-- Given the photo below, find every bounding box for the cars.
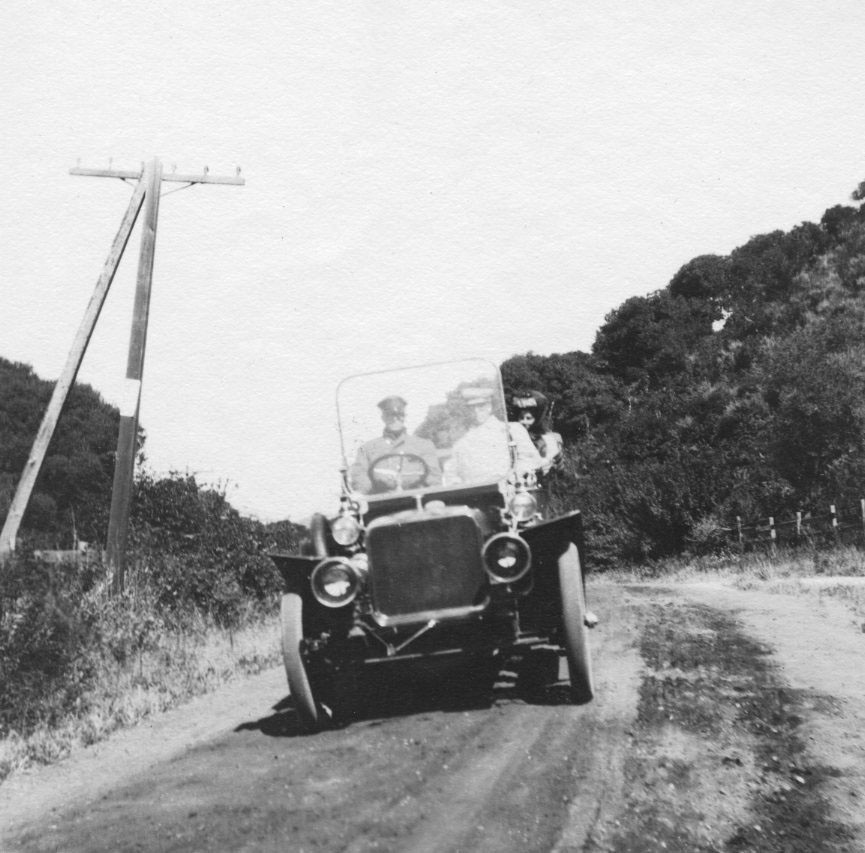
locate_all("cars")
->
[274,357,594,719]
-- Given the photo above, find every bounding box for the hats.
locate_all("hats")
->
[461,387,493,405]
[510,389,548,408]
[378,395,408,410]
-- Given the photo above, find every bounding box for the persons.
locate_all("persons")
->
[440,384,562,491]
[353,397,442,497]
